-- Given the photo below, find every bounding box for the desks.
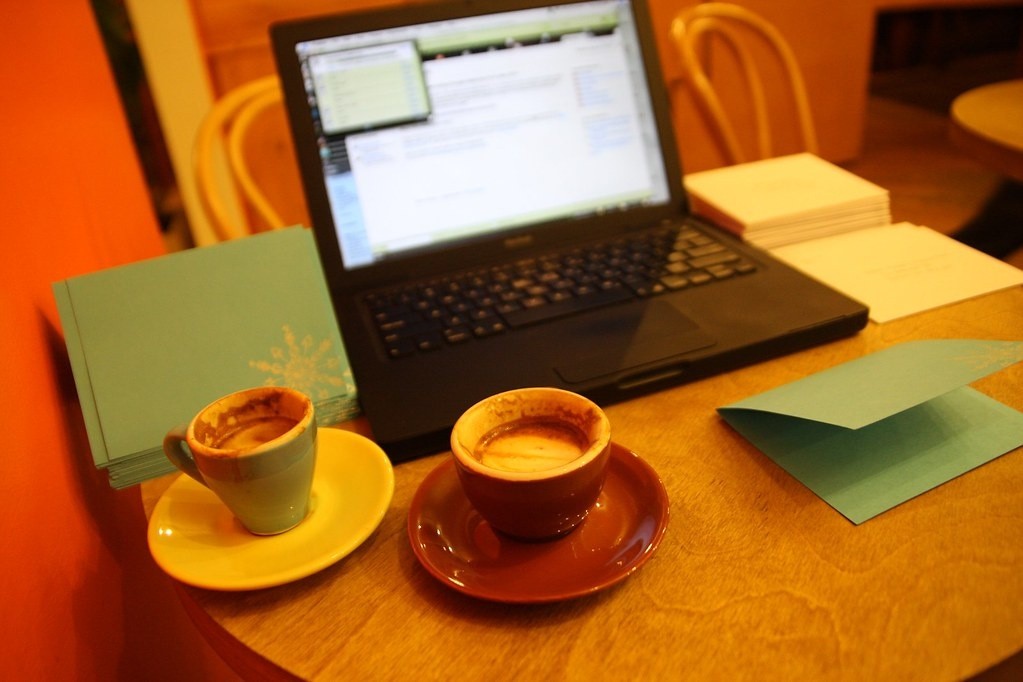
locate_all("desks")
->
[949,79,1023,181]
[137,287,1022,682]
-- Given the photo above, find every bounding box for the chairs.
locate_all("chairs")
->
[669,2,818,165]
[192,74,287,240]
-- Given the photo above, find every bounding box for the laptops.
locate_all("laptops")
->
[270,0,868,472]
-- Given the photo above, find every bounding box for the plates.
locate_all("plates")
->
[406,442,671,604]
[145,427,394,591]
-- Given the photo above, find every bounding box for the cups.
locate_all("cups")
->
[452,387,611,542]
[163,386,318,535]
